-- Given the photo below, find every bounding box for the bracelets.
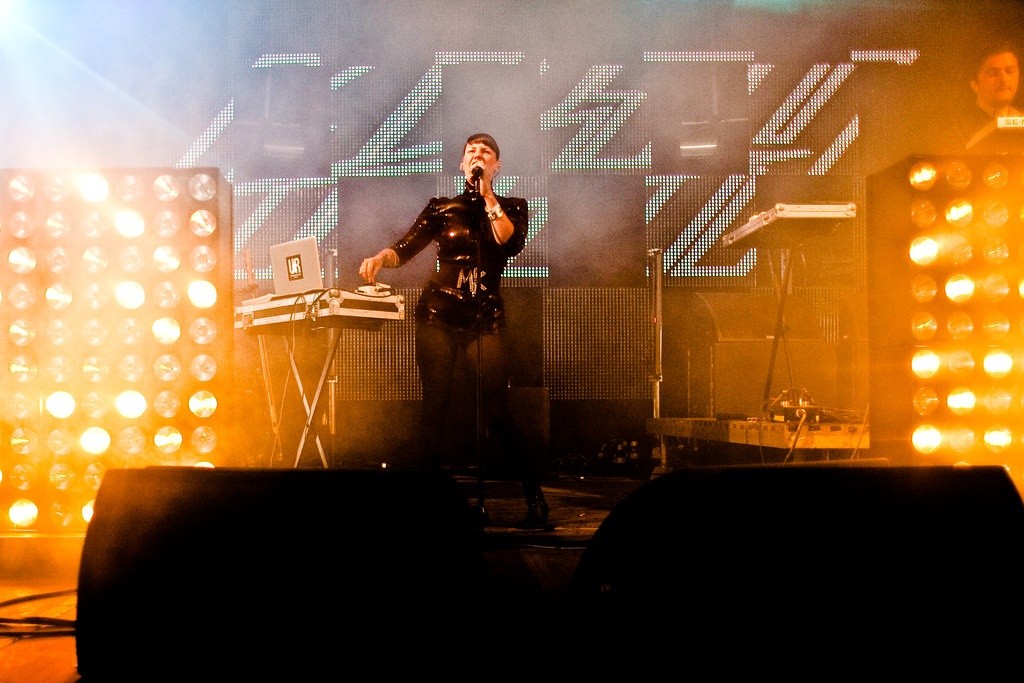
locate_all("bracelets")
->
[391,250,399,267]
[484,204,503,220]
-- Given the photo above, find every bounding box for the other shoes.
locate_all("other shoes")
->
[521,495,550,527]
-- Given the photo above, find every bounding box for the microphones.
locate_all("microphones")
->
[471,166,483,182]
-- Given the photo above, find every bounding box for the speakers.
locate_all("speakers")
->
[76,465,489,683]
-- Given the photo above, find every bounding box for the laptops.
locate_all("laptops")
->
[270,236,324,297]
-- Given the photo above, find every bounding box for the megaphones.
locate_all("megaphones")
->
[577,458,1024,683]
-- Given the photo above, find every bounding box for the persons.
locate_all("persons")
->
[358,132,551,529]
